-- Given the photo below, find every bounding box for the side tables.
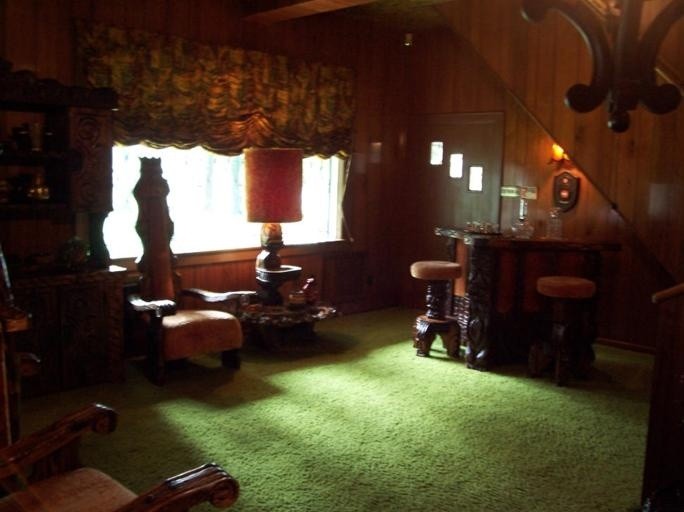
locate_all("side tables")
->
[239,298,344,358]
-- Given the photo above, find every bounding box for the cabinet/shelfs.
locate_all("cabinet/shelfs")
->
[0,53,129,400]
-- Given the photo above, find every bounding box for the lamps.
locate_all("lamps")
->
[243,147,303,307]
[547,143,570,170]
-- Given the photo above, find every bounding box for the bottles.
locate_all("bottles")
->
[510,186,535,240]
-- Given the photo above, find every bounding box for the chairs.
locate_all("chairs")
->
[0,401,240,512]
[123,157,251,388]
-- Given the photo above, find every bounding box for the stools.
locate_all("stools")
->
[528,275,598,387]
[408,260,462,358]
